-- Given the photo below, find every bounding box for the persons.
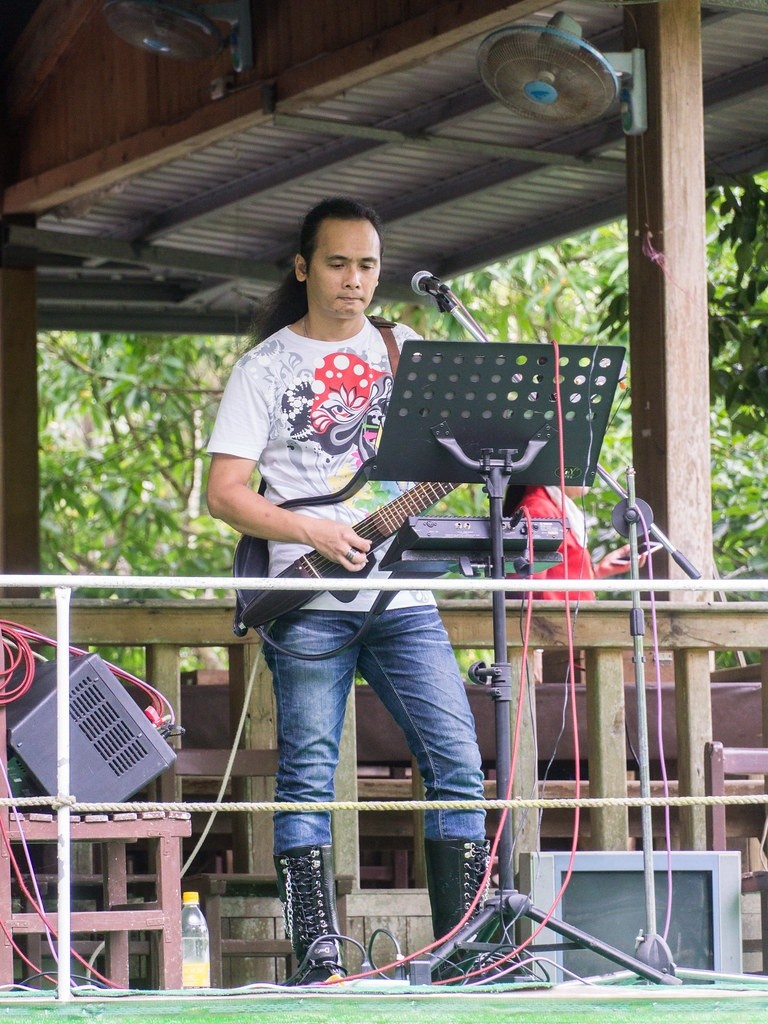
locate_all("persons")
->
[206,197,532,984]
[500,471,645,602]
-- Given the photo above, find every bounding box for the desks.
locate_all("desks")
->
[0,599,768,977]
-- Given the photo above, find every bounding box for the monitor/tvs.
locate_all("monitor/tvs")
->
[514,852,743,984]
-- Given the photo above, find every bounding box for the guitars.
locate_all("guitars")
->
[232,358,629,661]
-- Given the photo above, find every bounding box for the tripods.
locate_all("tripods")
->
[369,276,767,990]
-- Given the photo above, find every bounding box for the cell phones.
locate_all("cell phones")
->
[611,540,663,565]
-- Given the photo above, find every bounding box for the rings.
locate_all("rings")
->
[344,547,356,561]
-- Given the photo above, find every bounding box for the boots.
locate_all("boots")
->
[424,838,511,981]
[270,844,352,983]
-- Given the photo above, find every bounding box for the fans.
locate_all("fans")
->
[474,12,651,138]
[100,0,280,119]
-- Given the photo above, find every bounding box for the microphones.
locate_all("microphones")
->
[410,270,449,295]
